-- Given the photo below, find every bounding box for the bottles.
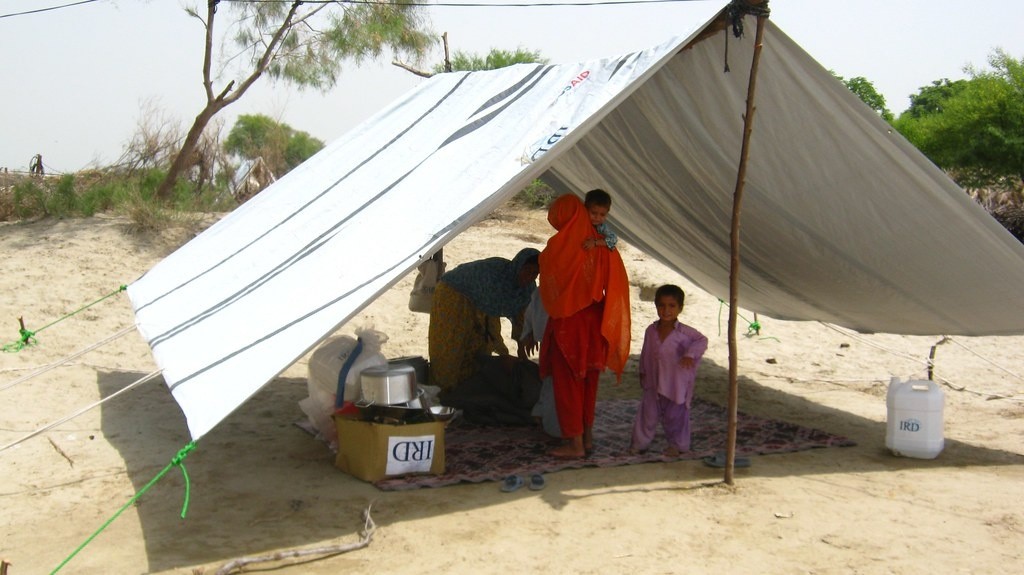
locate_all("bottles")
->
[885,377,945,459]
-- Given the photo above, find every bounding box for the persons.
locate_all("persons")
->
[580,189,619,252]
[520,192,630,457]
[623,283,708,455]
[426,248,541,399]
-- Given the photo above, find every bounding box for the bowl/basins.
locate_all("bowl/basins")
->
[427,406,455,421]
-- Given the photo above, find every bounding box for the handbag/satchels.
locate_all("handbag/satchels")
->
[409,244,447,314]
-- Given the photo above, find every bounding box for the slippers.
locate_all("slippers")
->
[703,453,749,469]
[500,473,525,492]
[527,472,545,491]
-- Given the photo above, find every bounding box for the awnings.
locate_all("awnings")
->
[211,156,278,209]
[1,0,1024,574]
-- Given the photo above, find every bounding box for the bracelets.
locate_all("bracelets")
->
[594,239,597,247]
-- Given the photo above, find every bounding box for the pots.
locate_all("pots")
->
[355,363,423,407]
